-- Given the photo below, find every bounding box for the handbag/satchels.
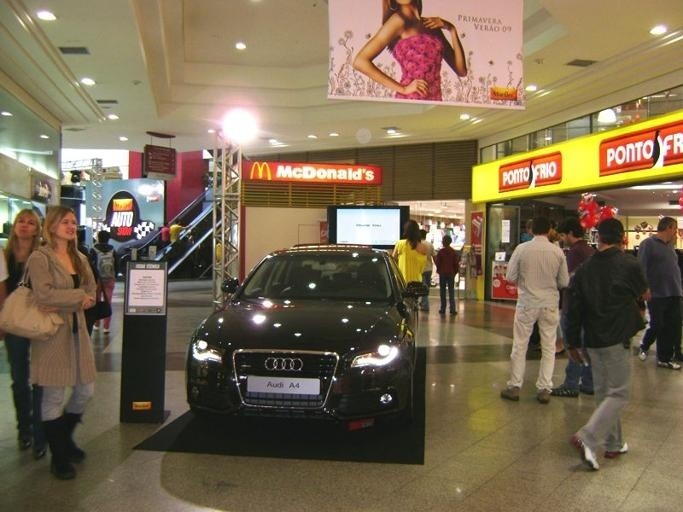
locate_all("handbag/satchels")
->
[94,301,112,321]
[0,249,66,343]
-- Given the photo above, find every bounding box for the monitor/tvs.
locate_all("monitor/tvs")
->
[328,206,410,250]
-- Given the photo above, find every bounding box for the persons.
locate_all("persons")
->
[560,218,649,472]
[418,229,436,312]
[160,220,170,245]
[435,234,459,315]
[351,0,467,101]
[391,219,429,286]
[501,211,683,403]
[169,219,189,243]
[0,203,118,480]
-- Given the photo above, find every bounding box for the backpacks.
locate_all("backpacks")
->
[91,246,116,279]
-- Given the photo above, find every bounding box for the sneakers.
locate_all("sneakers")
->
[419,306,458,316]
[93,324,111,333]
[499,386,521,402]
[656,350,683,370]
[603,439,628,459]
[578,384,595,396]
[536,387,551,404]
[570,430,602,473]
[638,344,648,361]
[549,385,580,397]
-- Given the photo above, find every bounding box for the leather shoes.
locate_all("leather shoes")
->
[528,340,567,357]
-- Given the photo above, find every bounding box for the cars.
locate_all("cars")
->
[177,238,431,438]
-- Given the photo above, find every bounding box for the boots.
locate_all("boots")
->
[40,416,77,481]
[12,386,33,451]
[62,412,86,464]
[30,384,47,461]
